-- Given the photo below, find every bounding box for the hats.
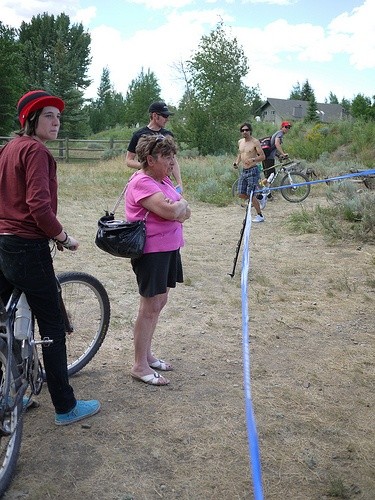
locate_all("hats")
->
[149,101,174,116]
[17,90,65,128]
[282,122,291,127]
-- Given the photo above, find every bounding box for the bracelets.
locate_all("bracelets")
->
[252,157,255,162]
[55,232,67,243]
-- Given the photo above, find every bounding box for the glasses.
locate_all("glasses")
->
[241,128,249,132]
[285,126,290,129]
[149,135,164,155]
[157,113,169,118]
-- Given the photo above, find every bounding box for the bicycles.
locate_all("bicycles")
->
[0,239,110,500]
[232,157,311,202]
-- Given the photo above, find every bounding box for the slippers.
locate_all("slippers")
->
[130,370,169,386]
[148,360,173,371]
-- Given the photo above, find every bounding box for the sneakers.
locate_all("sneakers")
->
[55,399,101,426]
[0,396,33,409]
[251,215,264,223]
[260,194,267,210]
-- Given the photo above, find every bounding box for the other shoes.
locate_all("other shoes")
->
[267,196,278,201]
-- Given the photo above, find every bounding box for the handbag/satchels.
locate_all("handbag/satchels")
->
[257,136,271,156]
[94,170,151,258]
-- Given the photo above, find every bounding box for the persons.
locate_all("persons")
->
[259,121,291,200]
[0,91,101,425]
[233,123,267,223]
[126,101,182,194]
[125,133,191,386]
